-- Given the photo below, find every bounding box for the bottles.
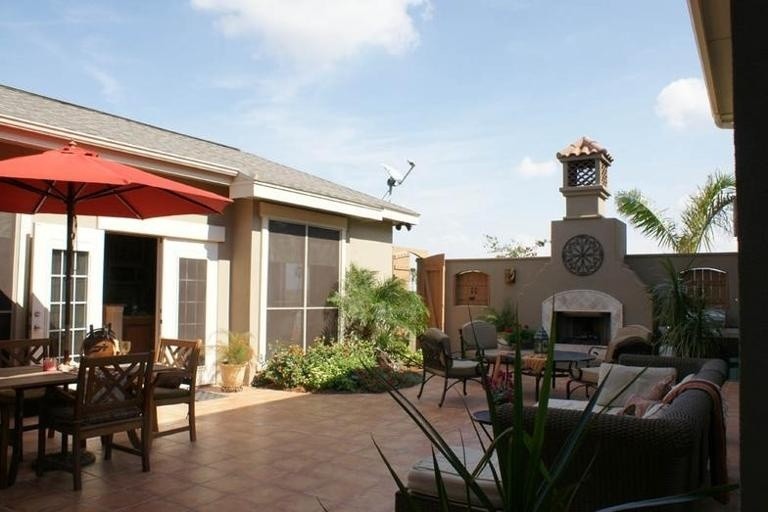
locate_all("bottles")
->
[43,356,57,370]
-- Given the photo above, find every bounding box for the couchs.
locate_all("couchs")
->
[393,354,728,511]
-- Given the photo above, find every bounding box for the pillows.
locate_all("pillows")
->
[596,363,675,408]
[154,368,186,390]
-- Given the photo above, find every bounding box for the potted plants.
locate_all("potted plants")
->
[204,331,256,393]
[471,293,524,350]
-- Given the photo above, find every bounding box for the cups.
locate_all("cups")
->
[120,340,132,355]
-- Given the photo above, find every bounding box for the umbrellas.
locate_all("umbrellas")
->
[0,139,236,365]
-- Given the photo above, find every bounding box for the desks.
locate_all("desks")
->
[471,410,492,445]
[0,360,176,473]
[496,349,596,402]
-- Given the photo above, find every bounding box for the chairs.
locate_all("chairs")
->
[588,324,653,358]
[36,350,158,491]
[567,336,652,399]
[414,328,490,407]
[458,321,515,391]
[0,338,86,463]
[133,338,201,442]
